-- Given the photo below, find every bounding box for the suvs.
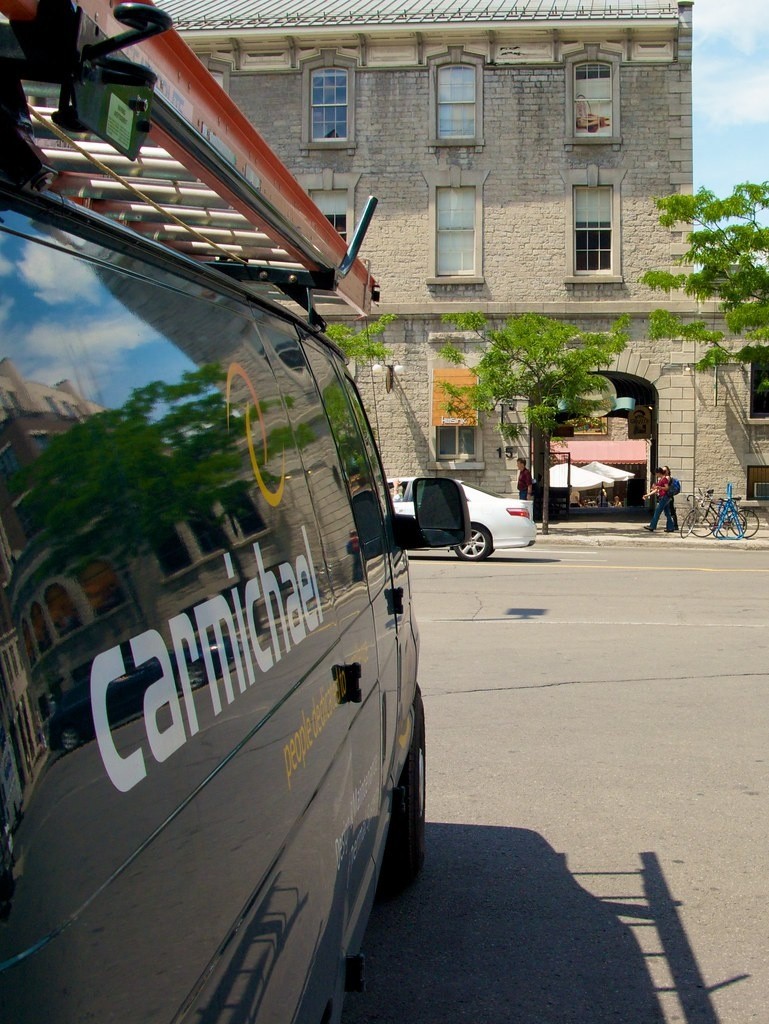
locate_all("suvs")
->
[0,1,474,1024]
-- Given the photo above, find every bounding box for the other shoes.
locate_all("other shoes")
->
[645,526,654,532]
[664,528,674,532]
[675,526,679,529]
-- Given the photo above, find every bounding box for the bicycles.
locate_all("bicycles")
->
[680,489,760,539]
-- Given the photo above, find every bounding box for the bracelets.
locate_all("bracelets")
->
[527,493,531,495]
[394,489,398,490]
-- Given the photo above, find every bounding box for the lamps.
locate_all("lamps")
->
[372,360,405,393]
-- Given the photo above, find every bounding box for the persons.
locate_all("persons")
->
[570,491,582,507]
[516,458,532,499]
[393,479,407,501]
[642,466,679,532]
[597,489,608,507]
[613,496,622,507]
[347,530,364,583]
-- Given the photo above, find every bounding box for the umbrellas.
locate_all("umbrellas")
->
[549,464,614,491]
[578,460,635,481]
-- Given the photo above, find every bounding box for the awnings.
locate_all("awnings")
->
[550,439,648,465]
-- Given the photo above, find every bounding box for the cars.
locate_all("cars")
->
[386,477,536,562]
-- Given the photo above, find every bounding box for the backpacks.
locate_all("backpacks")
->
[664,476,680,496]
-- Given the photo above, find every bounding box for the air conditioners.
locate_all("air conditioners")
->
[754,482,769,498]
